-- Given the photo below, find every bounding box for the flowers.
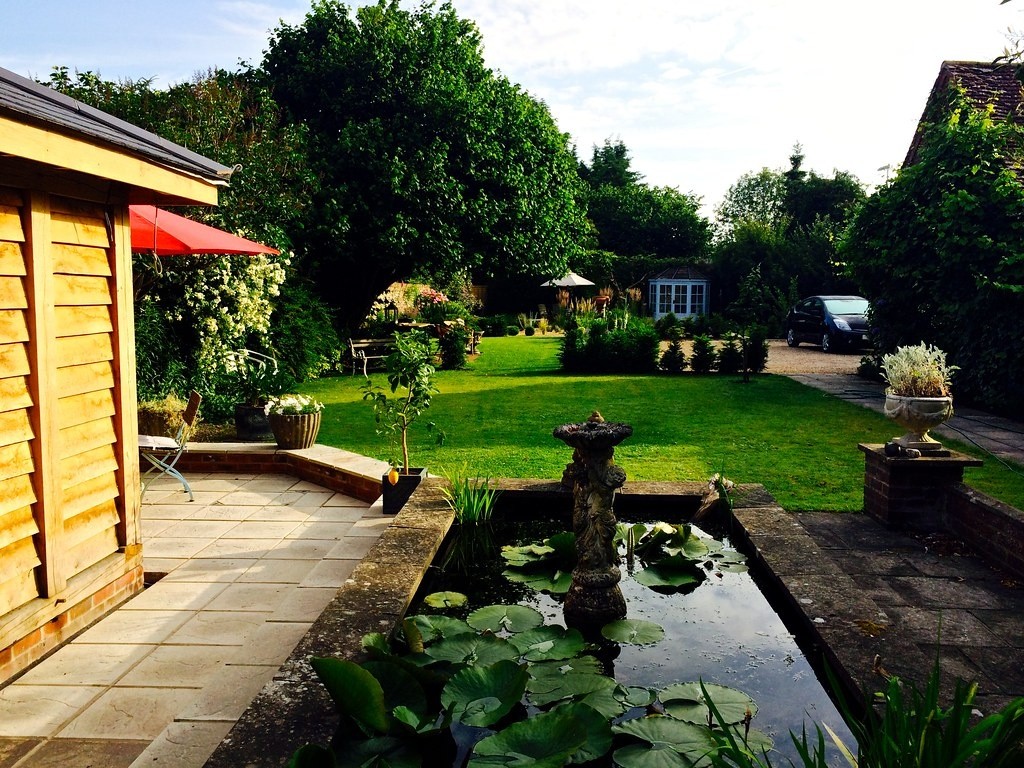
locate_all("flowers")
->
[264,394,326,416]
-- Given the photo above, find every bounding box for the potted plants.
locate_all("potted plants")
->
[358,328,446,514]
[138,390,202,437]
[878,339,959,448]
[464,315,485,354]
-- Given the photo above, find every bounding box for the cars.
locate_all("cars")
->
[783,295,876,353]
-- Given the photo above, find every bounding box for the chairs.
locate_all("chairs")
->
[223,349,281,402]
[138,389,203,507]
[433,324,452,362]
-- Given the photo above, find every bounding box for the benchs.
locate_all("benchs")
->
[347,337,397,379]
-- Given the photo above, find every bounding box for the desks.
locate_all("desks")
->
[395,320,435,344]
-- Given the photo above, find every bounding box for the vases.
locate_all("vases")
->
[269,412,322,449]
[236,403,273,440]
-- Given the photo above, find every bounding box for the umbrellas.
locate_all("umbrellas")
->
[541,271,595,311]
[128,205,280,256]
